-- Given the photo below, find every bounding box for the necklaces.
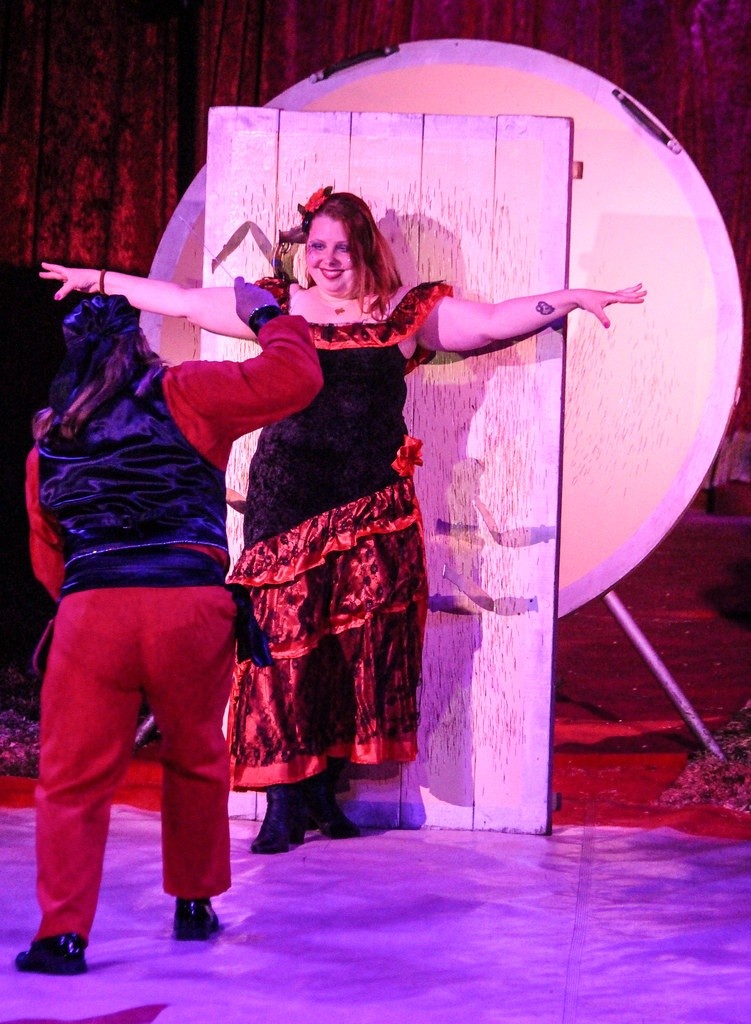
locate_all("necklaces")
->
[322,299,356,315]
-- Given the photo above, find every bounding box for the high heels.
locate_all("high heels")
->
[252,783,306,854]
[297,773,360,839]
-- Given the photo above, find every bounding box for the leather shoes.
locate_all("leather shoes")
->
[173,893,216,941]
[15,927,87,976]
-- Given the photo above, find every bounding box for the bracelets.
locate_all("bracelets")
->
[99,269,106,294]
[247,303,279,325]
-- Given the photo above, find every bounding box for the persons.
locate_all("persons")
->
[14,277,322,977]
[39,186,646,855]
